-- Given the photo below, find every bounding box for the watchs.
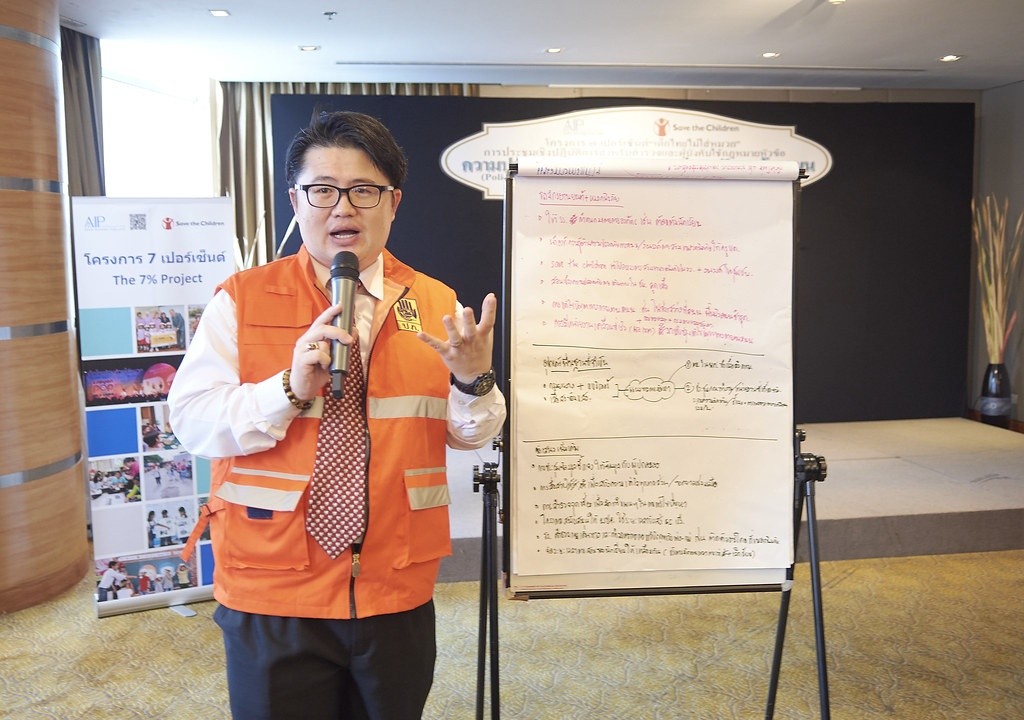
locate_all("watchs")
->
[450,366,497,397]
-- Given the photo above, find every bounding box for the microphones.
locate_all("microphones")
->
[330,251,360,398]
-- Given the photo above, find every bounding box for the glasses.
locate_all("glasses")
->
[295,183,394,208]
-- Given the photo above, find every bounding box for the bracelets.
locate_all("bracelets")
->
[283,370,315,409]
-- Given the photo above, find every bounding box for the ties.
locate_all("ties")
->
[305,277,367,559]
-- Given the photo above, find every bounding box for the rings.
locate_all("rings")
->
[307,342,319,351]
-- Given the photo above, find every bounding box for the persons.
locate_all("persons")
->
[136,309,185,352]
[168,110,507,720]
[149,507,191,546]
[192,314,201,333]
[143,422,163,451]
[91,457,141,502]
[98,561,190,600]
[152,467,162,485]
[169,460,187,482]
